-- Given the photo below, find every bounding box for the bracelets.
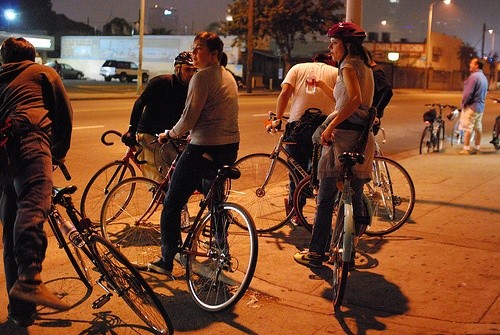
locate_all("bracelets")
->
[164,129,170,140]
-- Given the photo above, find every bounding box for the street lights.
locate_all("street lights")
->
[423,0,451,92]
[486,28,496,91]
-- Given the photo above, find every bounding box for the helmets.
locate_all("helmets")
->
[326,21,367,38]
[173,52,194,66]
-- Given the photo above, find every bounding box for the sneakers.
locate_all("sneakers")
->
[294,252,322,268]
[150,258,172,277]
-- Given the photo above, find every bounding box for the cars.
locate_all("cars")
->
[43,62,86,80]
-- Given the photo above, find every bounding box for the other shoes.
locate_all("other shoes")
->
[11,280,71,311]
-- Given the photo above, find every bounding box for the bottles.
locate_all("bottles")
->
[57,216,85,247]
[304,68,317,95]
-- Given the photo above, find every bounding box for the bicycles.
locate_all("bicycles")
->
[44,157,174,335]
[99,128,261,313]
[223,112,417,237]
[81,127,234,229]
[487,96,500,153]
[294,132,376,312]
[369,124,402,221]
[420,102,457,154]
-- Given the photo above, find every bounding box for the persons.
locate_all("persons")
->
[457,56,489,156]
[265,52,338,227]
[147,29,241,277]
[119,50,200,236]
[364,48,394,136]
[0,35,74,327]
[293,21,377,270]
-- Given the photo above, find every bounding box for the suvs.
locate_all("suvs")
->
[100,59,152,83]
